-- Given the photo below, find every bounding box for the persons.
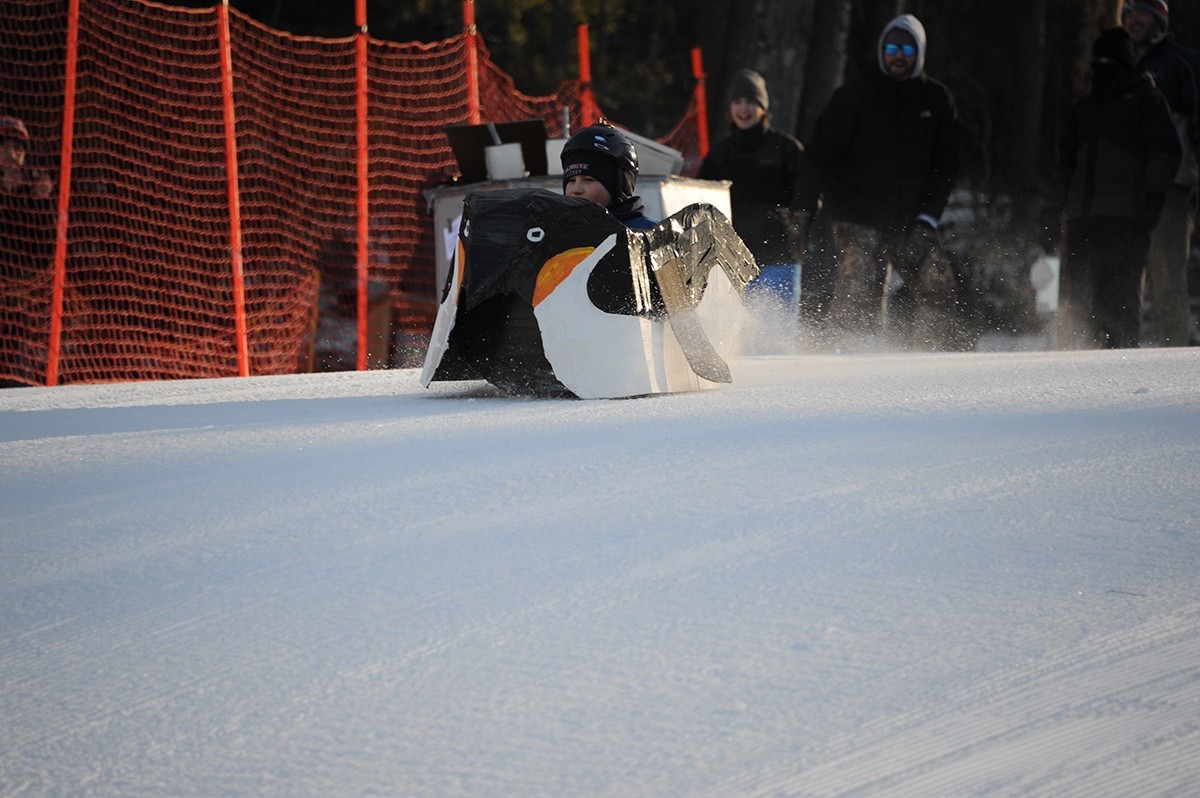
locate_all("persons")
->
[695,67,817,343]
[796,7,969,358]
[0,115,60,394]
[1036,23,1187,352]
[559,116,659,239]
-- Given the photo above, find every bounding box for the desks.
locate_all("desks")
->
[423,171,733,309]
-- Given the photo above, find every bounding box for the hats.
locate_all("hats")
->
[730,71,770,111]
[1087,26,1135,61]
[560,153,635,203]
[884,28,917,44]
[1,116,28,144]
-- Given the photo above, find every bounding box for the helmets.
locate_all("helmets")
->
[1121,0,1169,37]
[560,122,642,173]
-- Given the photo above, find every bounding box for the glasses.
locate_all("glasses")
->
[884,41,915,57]
